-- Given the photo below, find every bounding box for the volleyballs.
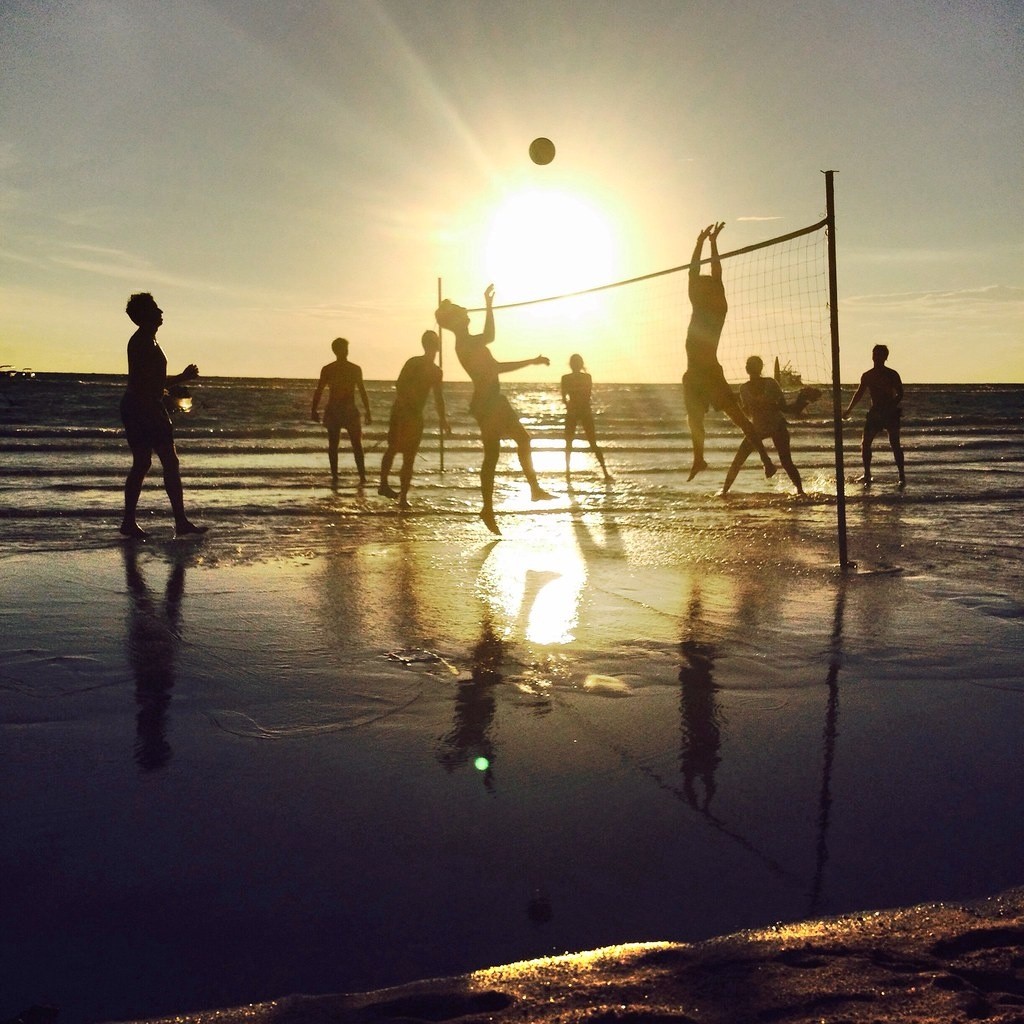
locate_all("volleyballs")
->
[529,137,557,166]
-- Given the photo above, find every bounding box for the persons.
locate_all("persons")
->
[378,331,451,507]
[682,222,777,482]
[312,338,372,483]
[435,283,560,535]
[561,354,613,481]
[120,293,209,534]
[842,345,906,486]
[715,356,809,496]
